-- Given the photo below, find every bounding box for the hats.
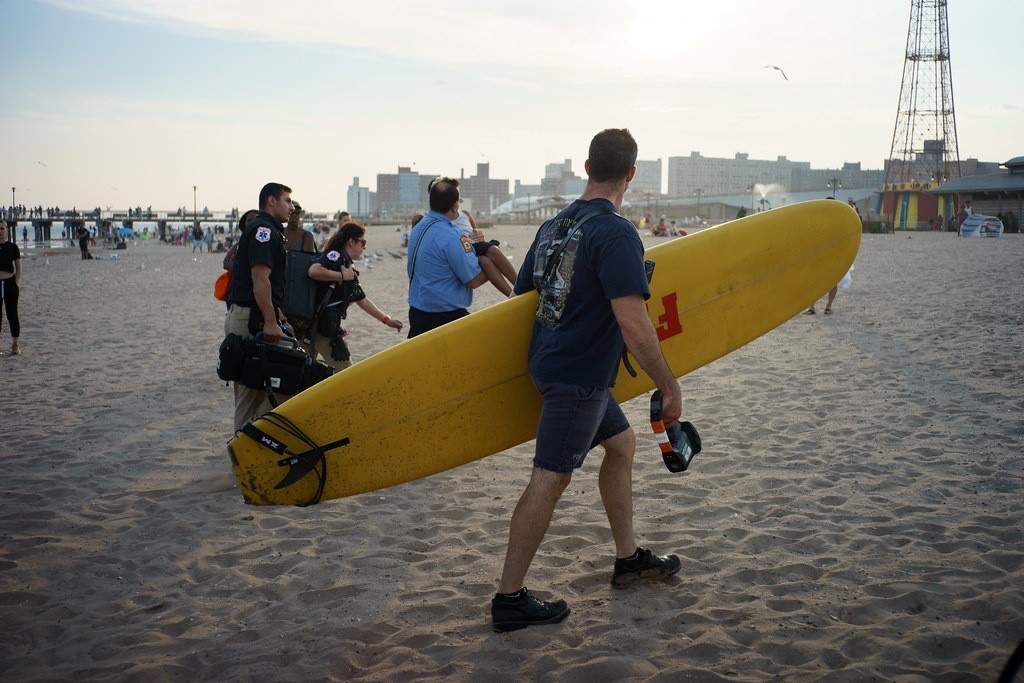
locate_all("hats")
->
[292,200,301,209]
[238,210,259,231]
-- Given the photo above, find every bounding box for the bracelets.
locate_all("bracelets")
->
[281,318,288,324]
[340,271,344,280]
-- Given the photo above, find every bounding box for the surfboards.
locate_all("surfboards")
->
[227,198,863,509]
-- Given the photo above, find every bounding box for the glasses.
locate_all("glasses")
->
[0,227,4,230]
[357,239,367,246]
[292,210,301,214]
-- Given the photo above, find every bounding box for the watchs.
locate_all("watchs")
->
[382,315,391,324]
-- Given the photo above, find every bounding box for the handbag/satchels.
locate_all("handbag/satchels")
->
[214,270,231,300]
[223,241,239,270]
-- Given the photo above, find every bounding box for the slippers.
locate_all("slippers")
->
[806,309,815,314]
[824,309,833,314]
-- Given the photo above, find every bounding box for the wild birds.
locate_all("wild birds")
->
[761,65,789,81]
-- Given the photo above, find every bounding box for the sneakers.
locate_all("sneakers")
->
[610,547,682,590]
[491,587,571,633]
[12,341,21,355]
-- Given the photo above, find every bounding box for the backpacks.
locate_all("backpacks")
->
[286,249,345,340]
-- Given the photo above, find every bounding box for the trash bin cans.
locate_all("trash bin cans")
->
[862,221,891,233]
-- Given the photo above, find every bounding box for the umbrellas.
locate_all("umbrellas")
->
[117,228,133,242]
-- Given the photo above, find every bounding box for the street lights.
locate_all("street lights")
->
[826,175,842,200]
[643,191,654,214]
[692,188,705,218]
[12,187,16,208]
[746,183,756,215]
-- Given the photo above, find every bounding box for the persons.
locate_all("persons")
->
[89,225,94,246]
[803,198,837,316]
[296,223,403,374]
[77,223,90,260]
[637,213,678,238]
[192,222,214,253]
[215,222,244,252]
[92,226,97,246]
[428,177,518,297]
[231,206,240,219]
[168,225,191,248]
[489,128,682,632]
[408,177,501,341]
[0,220,21,355]
[310,211,351,253]
[0,204,153,220]
[225,182,296,434]
[956,200,973,236]
[284,200,314,253]
[23,226,28,242]
[140,228,150,244]
